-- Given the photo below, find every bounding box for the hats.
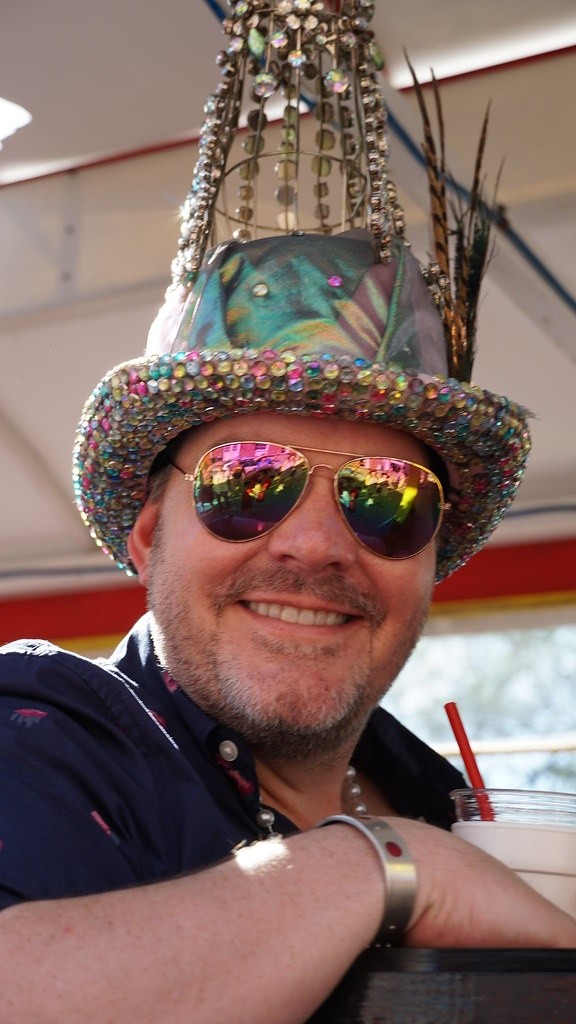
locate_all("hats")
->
[71,228,533,590]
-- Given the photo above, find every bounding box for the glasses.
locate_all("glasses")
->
[161,442,454,565]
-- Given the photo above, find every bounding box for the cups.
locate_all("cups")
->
[453,786,576,921]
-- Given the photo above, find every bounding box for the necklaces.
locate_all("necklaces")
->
[252,763,372,845]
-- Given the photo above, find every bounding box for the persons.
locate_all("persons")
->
[0,237,576,1023]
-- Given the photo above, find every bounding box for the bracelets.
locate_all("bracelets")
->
[314,813,420,950]
